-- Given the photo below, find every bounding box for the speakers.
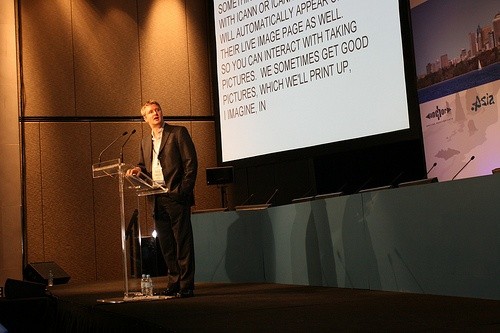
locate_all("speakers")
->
[4,261,70,300]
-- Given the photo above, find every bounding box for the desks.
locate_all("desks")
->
[191,173,500,300]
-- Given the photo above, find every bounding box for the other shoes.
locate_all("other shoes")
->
[154,286,180,295]
[176,289,193,299]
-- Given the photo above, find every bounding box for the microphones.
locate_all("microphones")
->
[451,156,475,180]
[98,129,136,167]
[266,189,278,204]
[391,173,403,185]
[424,163,437,179]
[242,194,254,206]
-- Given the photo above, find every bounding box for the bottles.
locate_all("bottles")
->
[48,271,53,286]
[141,274,153,297]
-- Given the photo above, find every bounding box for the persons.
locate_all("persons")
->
[125,99,199,299]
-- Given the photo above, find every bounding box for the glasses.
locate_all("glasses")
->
[143,108,162,116]
